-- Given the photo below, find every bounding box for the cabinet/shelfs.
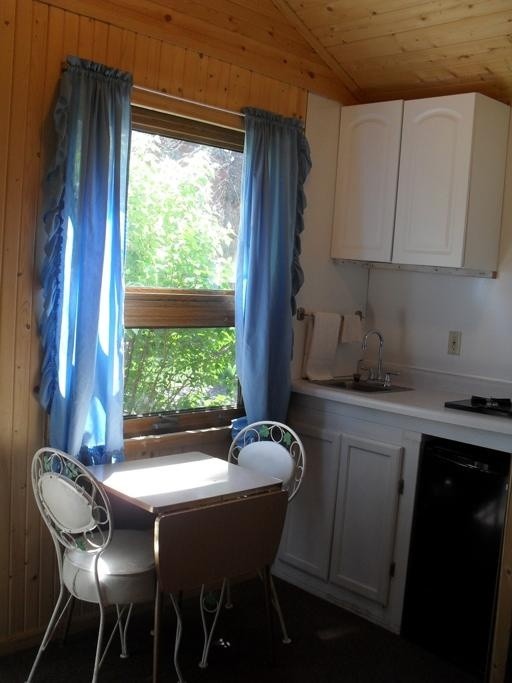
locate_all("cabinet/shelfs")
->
[279,420,405,607]
[329,91,510,272]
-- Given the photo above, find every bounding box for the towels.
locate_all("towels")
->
[338,313,363,343]
[300,311,342,382]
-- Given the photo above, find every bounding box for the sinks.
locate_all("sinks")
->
[309,379,413,393]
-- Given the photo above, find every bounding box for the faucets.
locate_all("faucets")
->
[362,328,385,380]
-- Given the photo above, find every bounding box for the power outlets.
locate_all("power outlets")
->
[448,330,462,353]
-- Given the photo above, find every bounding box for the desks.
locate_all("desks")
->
[75,451,285,513]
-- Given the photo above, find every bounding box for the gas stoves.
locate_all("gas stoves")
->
[442,393,512,422]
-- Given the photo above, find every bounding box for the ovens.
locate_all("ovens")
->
[390,434,512,677]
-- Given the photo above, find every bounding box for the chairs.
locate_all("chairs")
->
[151,420,306,667]
[26,446,183,683]
